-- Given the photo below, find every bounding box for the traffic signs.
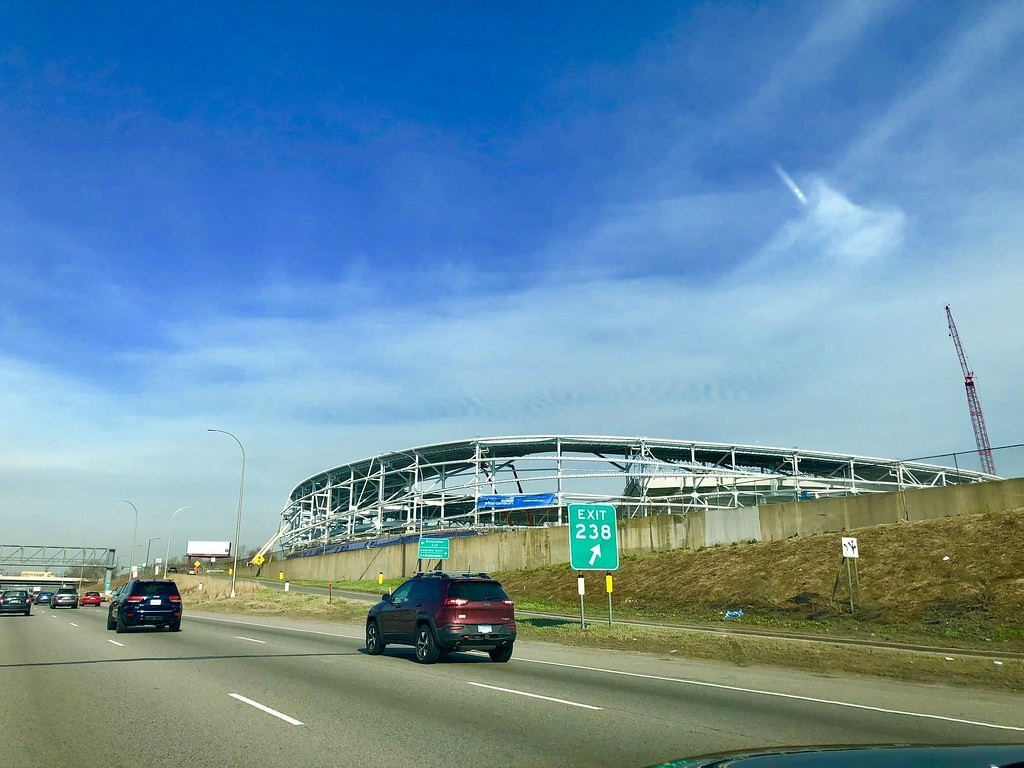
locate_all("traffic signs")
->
[567,502,619,572]
[418,537,451,560]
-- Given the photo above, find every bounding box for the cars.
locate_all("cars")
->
[0,588,44,603]
[79,591,102,606]
[34,591,54,605]
[187,568,197,576]
[0,590,35,616]
[168,566,178,574]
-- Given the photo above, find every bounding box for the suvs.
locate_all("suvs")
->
[363,570,518,666]
[49,587,79,609]
[107,577,184,634]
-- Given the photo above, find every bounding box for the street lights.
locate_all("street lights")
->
[163,505,198,579]
[206,428,246,598]
[115,554,128,572]
[389,451,424,574]
[115,498,138,581]
[144,537,161,574]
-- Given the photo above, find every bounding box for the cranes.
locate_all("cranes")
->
[944,305,996,474]
